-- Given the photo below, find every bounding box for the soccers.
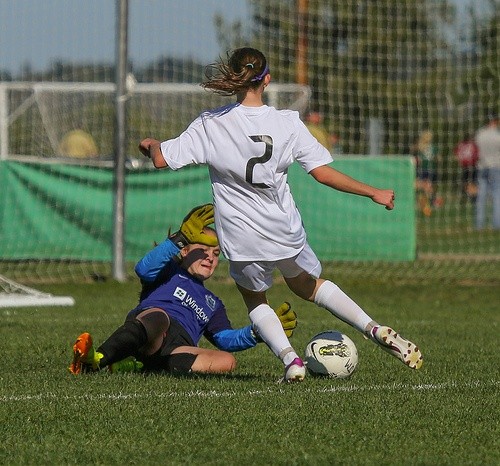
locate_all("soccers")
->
[305,331,358,379]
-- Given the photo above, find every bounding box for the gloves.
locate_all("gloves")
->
[267,299,298,336]
[170,203,218,250]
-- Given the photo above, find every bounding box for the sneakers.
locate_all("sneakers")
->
[109,356,145,374]
[69,333,104,375]
[284,358,306,383]
[371,324,423,370]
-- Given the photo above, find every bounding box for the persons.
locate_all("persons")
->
[57,119,100,161]
[472,111,500,230]
[454,134,480,204]
[137,47,423,384]
[69,204,298,377]
[409,128,444,215]
[304,109,337,149]
